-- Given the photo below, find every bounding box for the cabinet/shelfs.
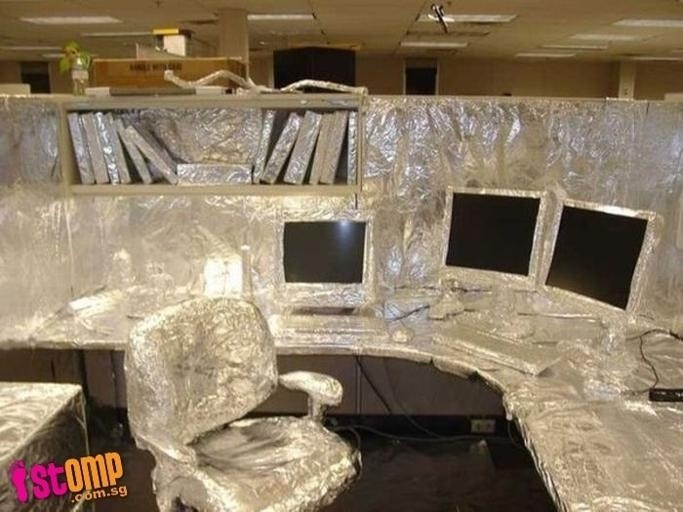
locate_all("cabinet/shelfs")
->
[56,98,367,194]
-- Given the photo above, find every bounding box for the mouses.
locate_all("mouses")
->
[390,326,415,343]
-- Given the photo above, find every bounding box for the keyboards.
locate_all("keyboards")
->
[267,313,383,334]
[433,322,563,374]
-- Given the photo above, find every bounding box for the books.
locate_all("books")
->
[63,111,360,188]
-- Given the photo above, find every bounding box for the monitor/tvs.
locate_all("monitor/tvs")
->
[440,184,547,292]
[540,197,664,321]
[274,208,374,310]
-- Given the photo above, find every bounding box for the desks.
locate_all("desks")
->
[0,262,680,507]
